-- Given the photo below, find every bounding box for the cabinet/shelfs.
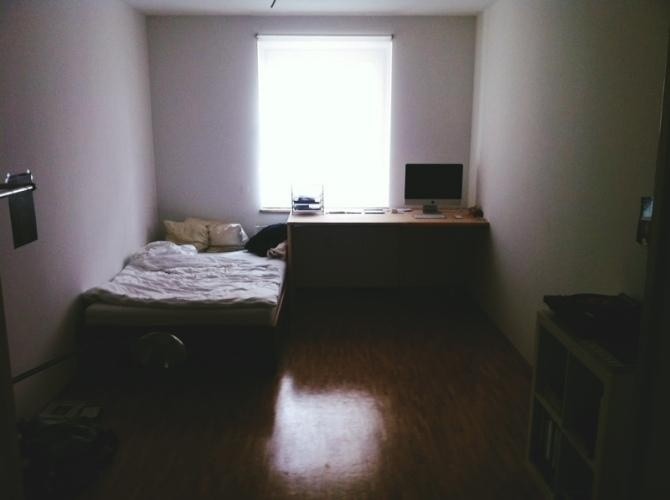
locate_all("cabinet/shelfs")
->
[524,292,648,500]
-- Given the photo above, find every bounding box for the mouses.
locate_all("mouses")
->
[455,215,463,219]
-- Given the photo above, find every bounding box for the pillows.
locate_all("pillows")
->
[163,220,208,252]
[187,217,225,234]
[209,221,250,252]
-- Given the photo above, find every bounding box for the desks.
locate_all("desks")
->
[286,207,489,313]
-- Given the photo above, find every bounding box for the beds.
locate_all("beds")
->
[86,238,287,417]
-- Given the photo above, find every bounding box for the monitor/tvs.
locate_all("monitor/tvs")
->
[403,162,464,206]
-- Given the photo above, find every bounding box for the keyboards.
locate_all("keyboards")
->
[414,214,445,218]
